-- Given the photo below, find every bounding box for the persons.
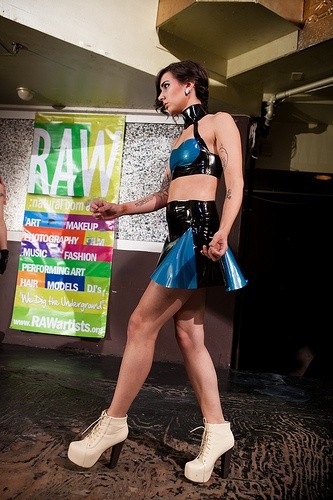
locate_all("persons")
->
[0,179,10,273]
[67,60,248,484]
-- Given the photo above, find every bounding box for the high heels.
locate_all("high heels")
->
[184,417,234,483]
[67,409,129,469]
